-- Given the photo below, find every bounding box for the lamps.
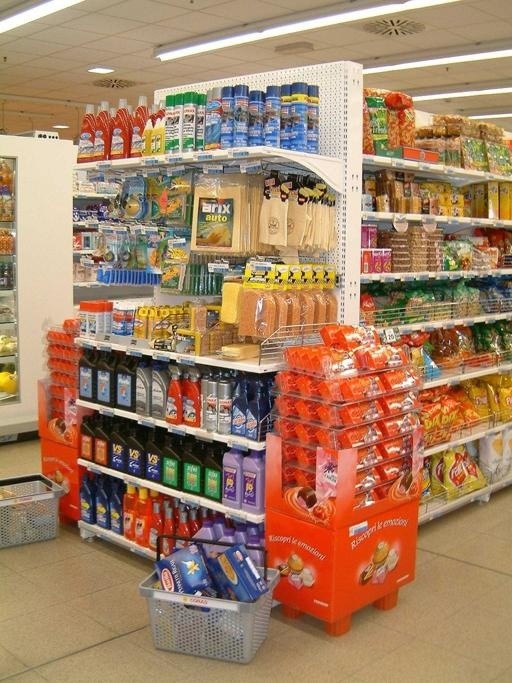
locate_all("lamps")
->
[468,112,512,122]
[0,0,87,35]
[407,85,512,103]
[151,0,463,64]
[363,40,512,74]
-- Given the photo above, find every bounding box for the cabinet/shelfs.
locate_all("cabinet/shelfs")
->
[74,60,352,609]
[357,85,512,529]
